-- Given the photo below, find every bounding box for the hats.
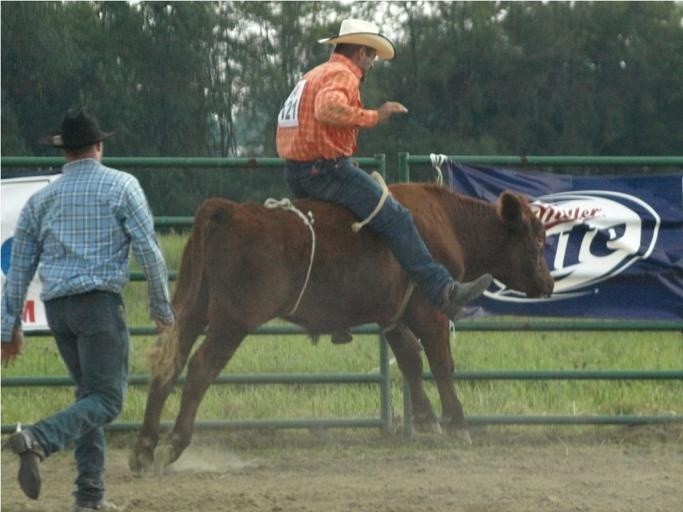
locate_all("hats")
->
[319,19,395,59]
[52,108,113,150]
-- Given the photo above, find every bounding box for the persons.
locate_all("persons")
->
[1,110,178,511]
[273,15,493,320]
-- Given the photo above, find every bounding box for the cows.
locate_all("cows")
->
[130,183,554,484]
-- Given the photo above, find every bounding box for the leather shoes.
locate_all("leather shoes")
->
[8,429,46,498]
[73,499,115,512]
[441,274,492,320]
[329,329,352,344]
